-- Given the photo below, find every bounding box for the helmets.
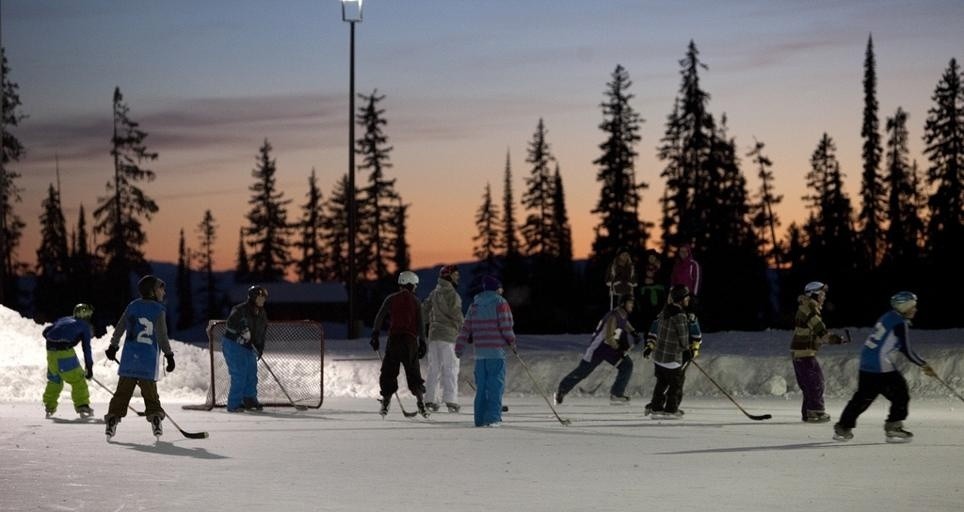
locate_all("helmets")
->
[891,291,917,314]
[672,285,689,302]
[74,304,93,318]
[137,275,156,297]
[248,285,268,297]
[439,265,458,277]
[398,271,420,285]
[618,293,634,306]
[804,282,824,297]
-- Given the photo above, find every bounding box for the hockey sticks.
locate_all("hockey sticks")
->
[114,357,209,440]
[251,342,308,411]
[579,345,636,395]
[512,349,572,426]
[823,329,852,345]
[92,377,145,416]
[375,349,418,417]
[460,370,508,412]
[691,359,772,421]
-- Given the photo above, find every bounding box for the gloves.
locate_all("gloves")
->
[369,337,379,350]
[418,340,426,358]
[164,353,175,371]
[85,366,93,379]
[922,364,934,376]
[618,331,700,359]
[242,329,251,343]
[828,335,841,344]
[105,345,119,360]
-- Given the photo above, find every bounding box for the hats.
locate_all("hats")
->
[481,275,503,291]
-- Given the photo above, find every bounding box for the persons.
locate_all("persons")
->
[41,300,98,419]
[369,269,432,417]
[833,291,935,440]
[100,275,175,436]
[553,292,643,404]
[218,284,271,413]
[604,243,703,421]
[420,264,466,412]
[789,281,844,423]
[455,274,519,428]
